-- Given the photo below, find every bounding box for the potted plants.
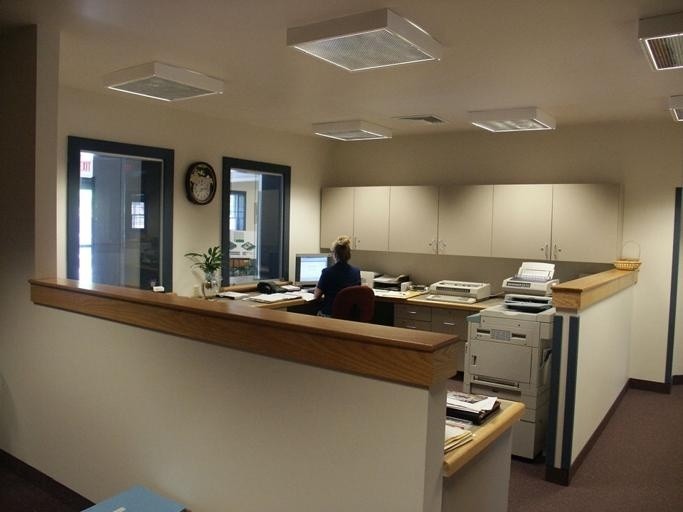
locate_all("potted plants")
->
[183,246,225,298]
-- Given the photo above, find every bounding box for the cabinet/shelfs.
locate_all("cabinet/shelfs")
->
[393,294,486,372]
[388,185,494,258]
[494,183,622,261]
[320,185,388,255]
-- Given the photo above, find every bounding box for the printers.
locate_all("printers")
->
[464,293,556,459]
[426,279,492,305]
[502,262,559,309]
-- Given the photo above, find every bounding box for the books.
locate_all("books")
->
[443,424,476,454]
[446,389,501,426]
[250,291,302,303]
[216,290,248,300]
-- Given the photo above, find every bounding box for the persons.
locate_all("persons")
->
[315,236,361,318]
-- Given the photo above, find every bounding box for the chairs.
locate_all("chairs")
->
[331,285,374,322]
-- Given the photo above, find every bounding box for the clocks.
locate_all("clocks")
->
[184,161,216,205]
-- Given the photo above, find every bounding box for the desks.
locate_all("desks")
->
[198,280,427,309]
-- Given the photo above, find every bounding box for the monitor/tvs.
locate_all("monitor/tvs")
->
[295,253,335,287]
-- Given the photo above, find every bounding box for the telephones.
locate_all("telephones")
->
[257,281,288,294]
